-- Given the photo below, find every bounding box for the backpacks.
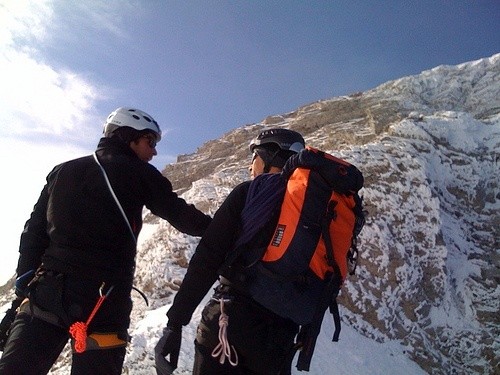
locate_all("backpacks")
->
[220,139,368,326]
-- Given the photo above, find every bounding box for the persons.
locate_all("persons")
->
[0,105,213,375]
[154,129,306,375]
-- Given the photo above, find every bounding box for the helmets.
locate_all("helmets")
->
[249,129,306,153]
[102,106,162,145]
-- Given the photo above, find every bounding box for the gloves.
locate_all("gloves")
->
[154,324,182,374]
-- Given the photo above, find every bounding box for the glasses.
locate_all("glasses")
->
[142,134,157,149]
[250,150,266,163]
